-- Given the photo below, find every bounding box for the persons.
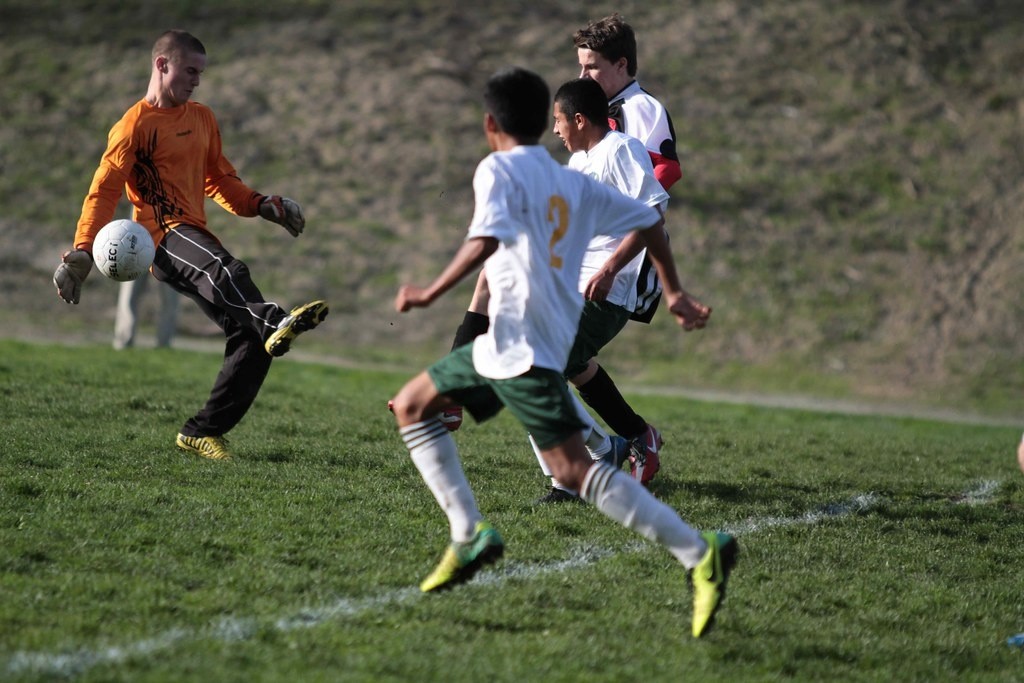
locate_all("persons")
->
[113,269,177,352]
[52,29,327,461]
[390,66,741,637]
[387,13,713,509]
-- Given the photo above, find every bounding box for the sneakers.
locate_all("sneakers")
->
[684,531,737,637]
[176,432,234,462]
[627,424,665,485]
[419,521,504,592]
[593,435,632,470]
[388,397,463,431]
[533,485,573,503]
[264,301,330,356]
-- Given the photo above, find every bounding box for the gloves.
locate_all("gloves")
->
[260,194,306,238]
[53,249,92,304]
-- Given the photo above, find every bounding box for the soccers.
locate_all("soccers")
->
[91,217,157,283]
